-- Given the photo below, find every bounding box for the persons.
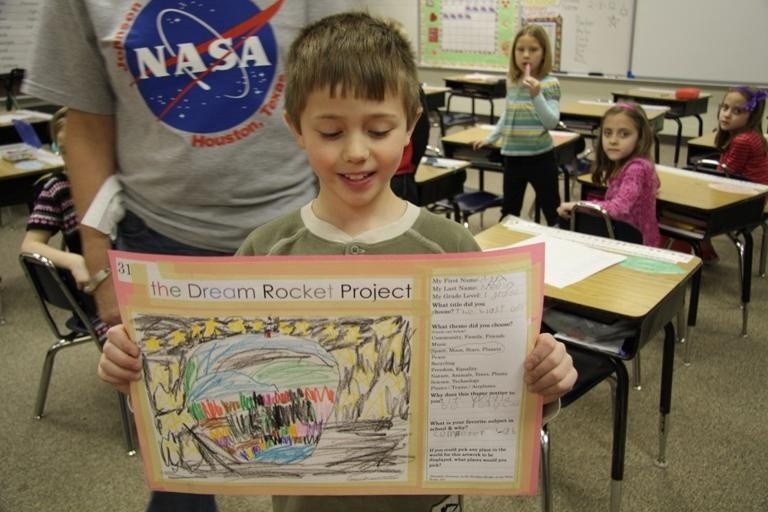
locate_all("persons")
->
[100,13,578,510]
[21,2,373,511]
[705,84,766,183]
[21,106,99,327]
[559,102,658,249]
[470,28,562,227]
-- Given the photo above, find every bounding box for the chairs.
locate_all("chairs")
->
[451,189,505,223]
[559,201,641,243]
[677,158,768,280]
[541,323,619,511]
[18,252,136,459]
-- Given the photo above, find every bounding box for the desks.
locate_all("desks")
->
[610,87,713,167]
[414,158,470,223]
[442,74,507,125]
[559,101,673,164]
[422,85,451,124]
[0,107,54,130]
[474,215,703,480]
[440,122,586,225]
[1,141,66,213]
[578,164,764,326]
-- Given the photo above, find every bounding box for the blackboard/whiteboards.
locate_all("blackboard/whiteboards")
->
[418,0,768,86]
